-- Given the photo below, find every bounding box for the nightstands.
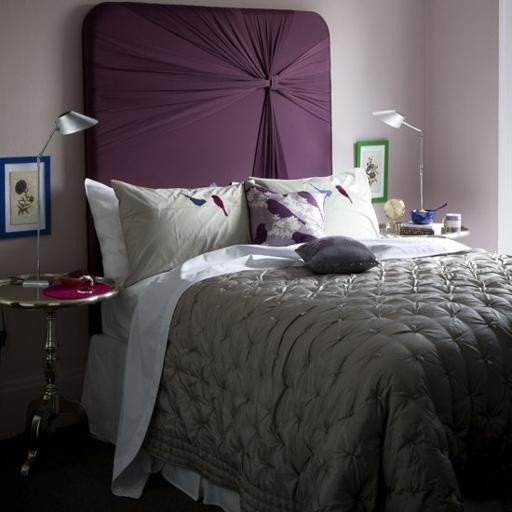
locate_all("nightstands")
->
[381,223,470,239]
[0,274,119,476]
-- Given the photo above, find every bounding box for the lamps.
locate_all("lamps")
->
[372,110,424,210]
[22,110,98,287]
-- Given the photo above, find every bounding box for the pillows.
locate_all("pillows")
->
[85,168,380,288]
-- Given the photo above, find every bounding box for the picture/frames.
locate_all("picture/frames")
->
[356,140,389,202]
[0,157,51,238]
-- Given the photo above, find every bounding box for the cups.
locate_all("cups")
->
[441,213,462,234]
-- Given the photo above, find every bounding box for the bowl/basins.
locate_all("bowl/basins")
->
[408,208,436,225]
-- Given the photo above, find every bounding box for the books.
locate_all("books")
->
[400,223,431,229]
[398,226,434,236]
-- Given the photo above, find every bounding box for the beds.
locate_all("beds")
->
[82,2,512,512]
